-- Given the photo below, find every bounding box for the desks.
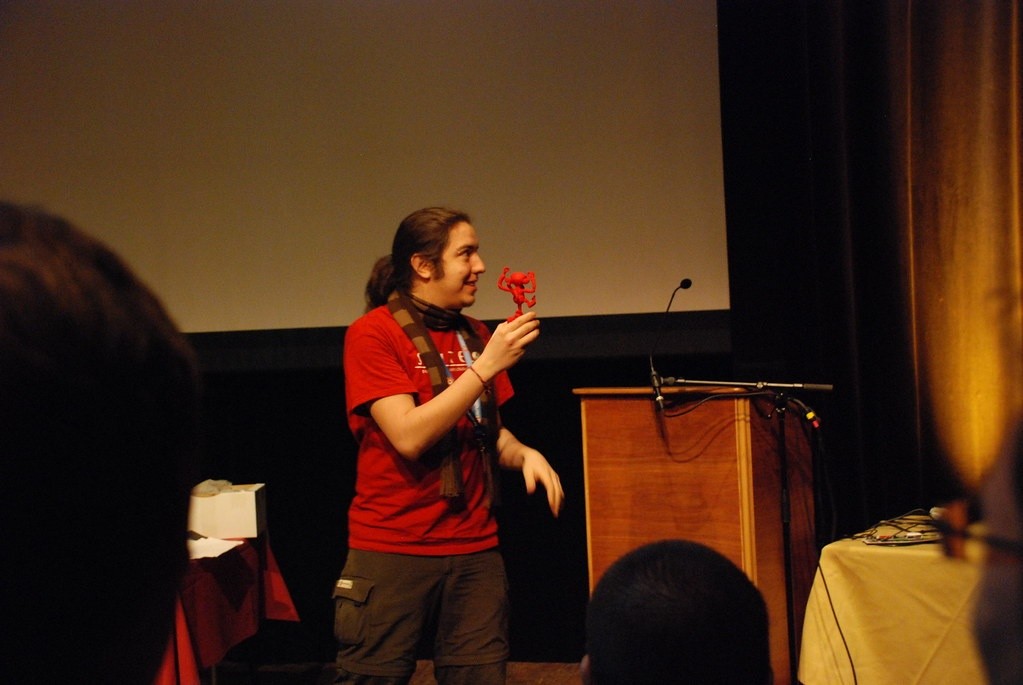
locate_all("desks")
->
[158,536,300,685]
[793,515,992,685]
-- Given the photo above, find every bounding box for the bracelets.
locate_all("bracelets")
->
[471,366,491,395]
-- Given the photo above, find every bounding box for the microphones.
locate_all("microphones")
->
[648,278,692,411]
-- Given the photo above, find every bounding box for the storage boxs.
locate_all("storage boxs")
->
[187,483,268,538]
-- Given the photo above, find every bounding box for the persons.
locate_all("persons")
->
[331,206,564,685]
[0,199,209,685]
[971,409,1023,685]
[578,540,774,685]
